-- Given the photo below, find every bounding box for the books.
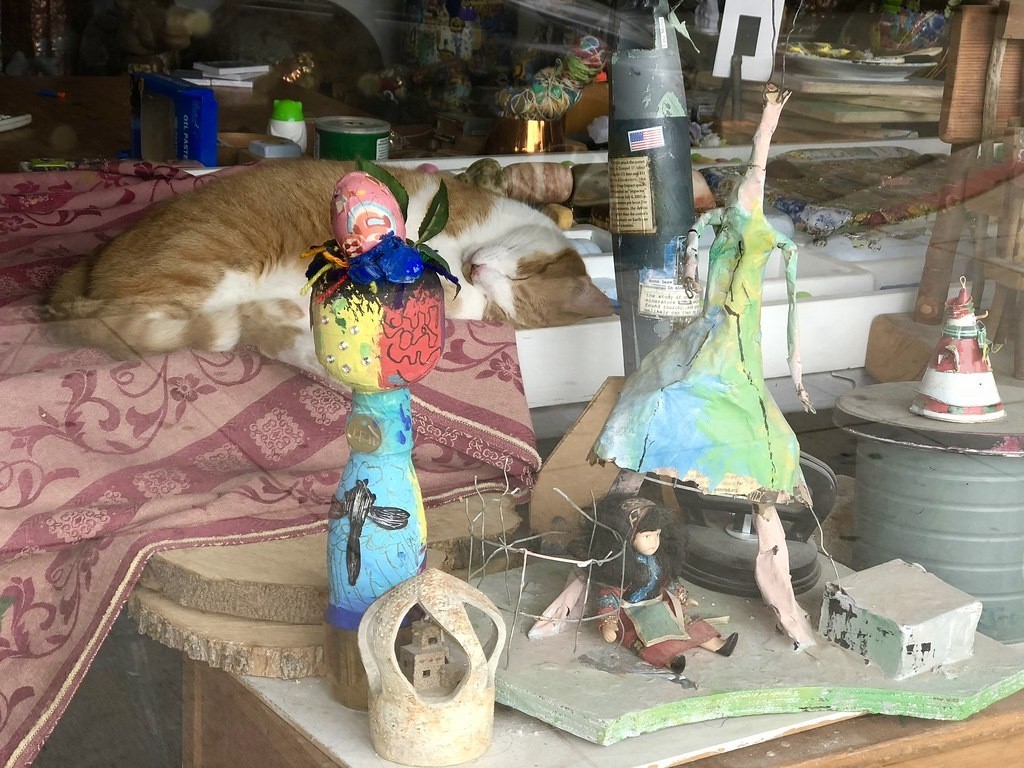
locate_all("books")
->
[0,109,32,132]
[743,71,944,139]
[172,58,269,88]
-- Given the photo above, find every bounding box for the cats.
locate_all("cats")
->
[41,156,612,377]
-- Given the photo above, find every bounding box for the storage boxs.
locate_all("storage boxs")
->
[129,72,218,169]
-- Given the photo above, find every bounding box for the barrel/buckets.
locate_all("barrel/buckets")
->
[313,115,392,160]
[852,437,1024,645]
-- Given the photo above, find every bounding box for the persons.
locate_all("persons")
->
[583,496,739,672]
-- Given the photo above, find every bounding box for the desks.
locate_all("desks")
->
[0,72,431,173]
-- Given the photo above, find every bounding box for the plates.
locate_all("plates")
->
[776,42,938,80]
